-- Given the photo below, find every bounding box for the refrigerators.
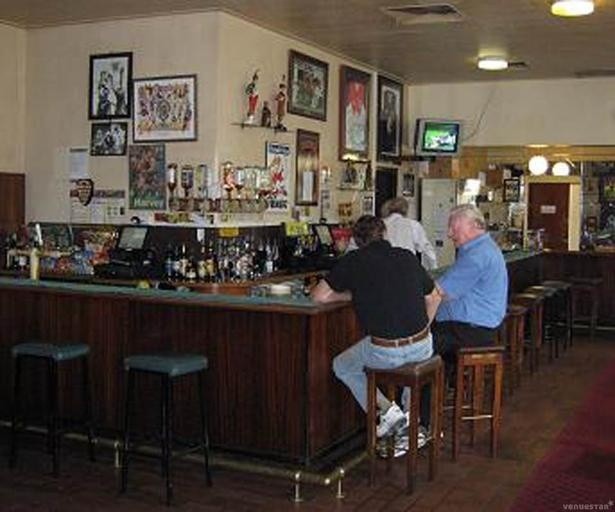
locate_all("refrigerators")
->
[419,174,467,271]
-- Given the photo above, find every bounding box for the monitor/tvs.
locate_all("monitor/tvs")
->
[313,224,334,257]
[416,119,462,156]
[113,225,149,262]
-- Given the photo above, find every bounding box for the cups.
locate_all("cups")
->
[245,279,307,302]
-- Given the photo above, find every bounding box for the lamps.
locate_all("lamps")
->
[477,54,509,71]
[553,145,570,176]
[551,1,595,17]
[526,145,548,175]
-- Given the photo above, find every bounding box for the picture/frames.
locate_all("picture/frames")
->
[375,74,404,160]
[89,122,128,156]
[89,51,133,120]
[335,61,371,162]
[131,73,197,143]
[295,127,321,207]
[286,49,329,121]
[503,178,520,202]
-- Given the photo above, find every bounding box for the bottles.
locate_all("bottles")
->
[164,159,270,215]
[161,232,281,282]
[3,219,30,273]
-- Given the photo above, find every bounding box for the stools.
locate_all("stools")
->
[365,352,444,492]
[492,272,604,396]
[117,349,212,504]
[10,339,101,472]
[445,343,506,461]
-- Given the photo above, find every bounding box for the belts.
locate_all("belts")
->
[370,328,430,348]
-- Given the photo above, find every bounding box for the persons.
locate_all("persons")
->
[422,203,511,438]
[346,79,367,150]
[298,140,317,201]
[245,68,264,120]
[274,74,288,121]
[131,146,157,188]
[307,214,441,457]
[600,206,615,230]
[344,195,439,271]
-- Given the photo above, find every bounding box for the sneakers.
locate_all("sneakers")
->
[375,400,427,458]
